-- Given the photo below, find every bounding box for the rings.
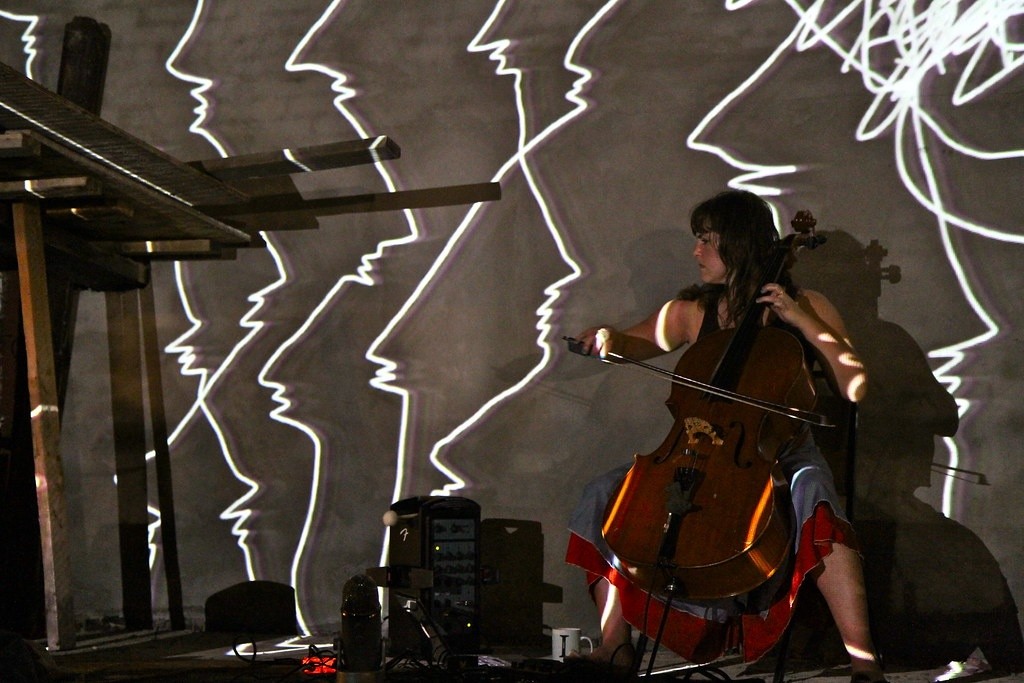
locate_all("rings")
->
[780,287,786,294]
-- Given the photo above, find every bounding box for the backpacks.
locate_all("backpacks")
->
[204,580,298,635]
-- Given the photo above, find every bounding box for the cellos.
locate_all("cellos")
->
[604,208,830,683]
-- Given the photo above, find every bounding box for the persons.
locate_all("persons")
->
[568,190,886,683]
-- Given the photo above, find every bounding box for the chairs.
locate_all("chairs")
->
[626,369,857,683]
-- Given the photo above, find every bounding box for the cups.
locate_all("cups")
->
[552,628,593,662]
